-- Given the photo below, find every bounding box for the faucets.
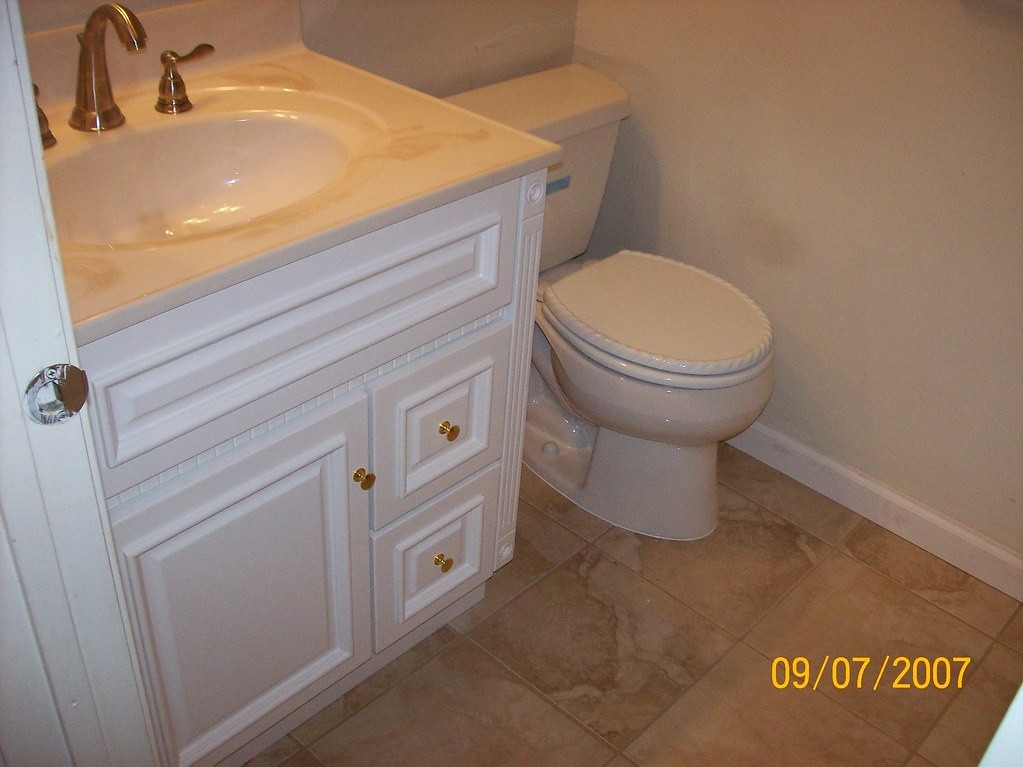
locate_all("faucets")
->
[68,3,147,133]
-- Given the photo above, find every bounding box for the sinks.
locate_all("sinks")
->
[46,87,392,253]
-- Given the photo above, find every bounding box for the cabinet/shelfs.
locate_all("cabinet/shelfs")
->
[77,166,549,767]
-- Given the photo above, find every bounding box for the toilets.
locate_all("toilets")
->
[442,63,775,541]
